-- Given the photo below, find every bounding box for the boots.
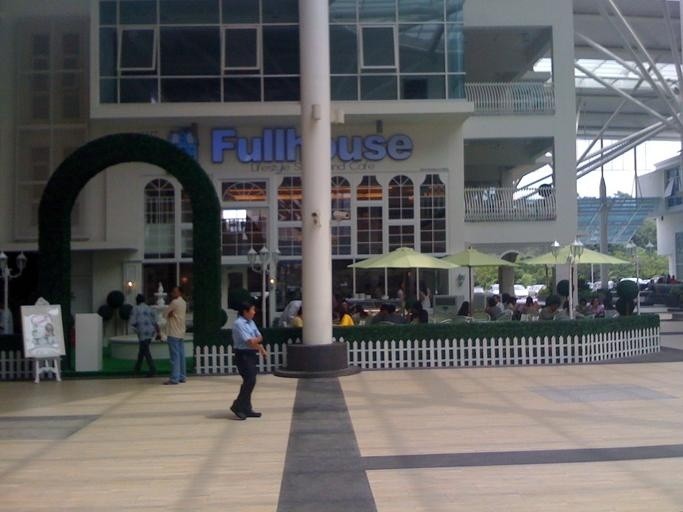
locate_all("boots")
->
[245,405,262,418]
[230,400,247,420]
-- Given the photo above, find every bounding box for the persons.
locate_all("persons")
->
[130,294,159,378]
[162,285,187,383]
[44,323,55,344]
[230,300,268,421]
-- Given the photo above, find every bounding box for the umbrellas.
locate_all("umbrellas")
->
[439,247,523,316]
[347,246,460,316]
[514,244,630,265]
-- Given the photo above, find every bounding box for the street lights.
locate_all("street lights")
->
[0,249,29,338]
[245,243,286,327]
[622,236,656,317]
[548,235,588,321]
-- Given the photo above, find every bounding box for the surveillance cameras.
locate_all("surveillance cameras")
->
[333,211,350,219]
[311,210,320,219]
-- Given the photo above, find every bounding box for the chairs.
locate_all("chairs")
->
[332,284,629,326]
[282,321,290,328]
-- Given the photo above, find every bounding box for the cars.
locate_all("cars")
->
[471,276,662,300]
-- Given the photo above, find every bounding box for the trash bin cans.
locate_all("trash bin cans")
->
[74,313,103,373]
